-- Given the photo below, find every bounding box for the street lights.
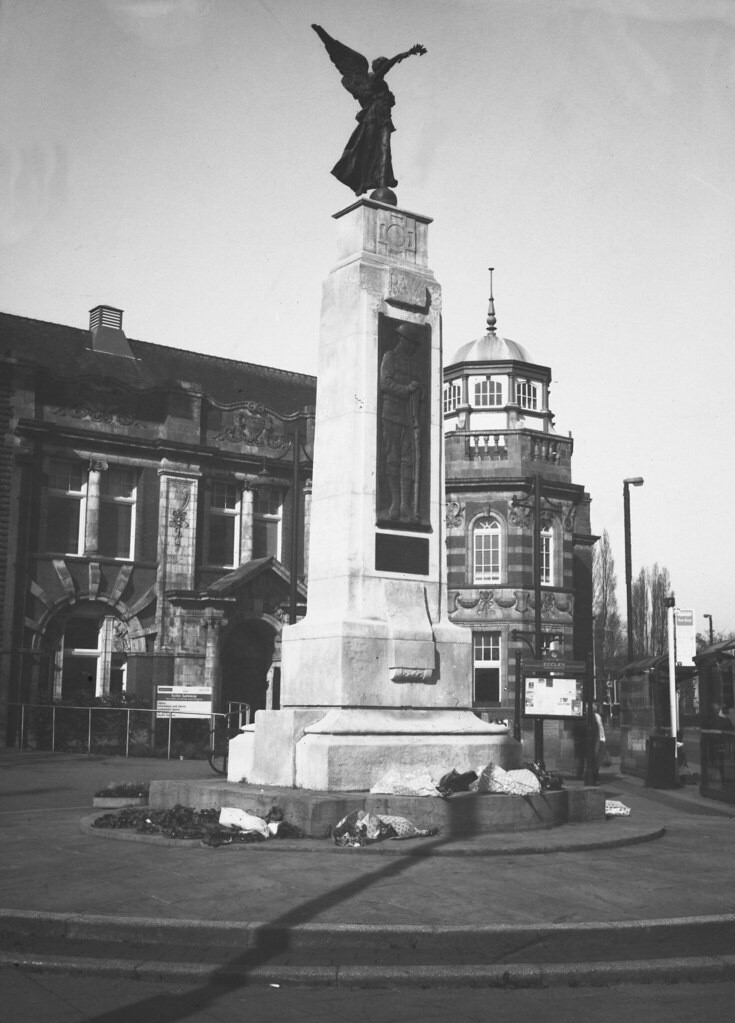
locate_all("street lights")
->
[508,472,568,789]
[621,476,644,665]
[703,614,713,647]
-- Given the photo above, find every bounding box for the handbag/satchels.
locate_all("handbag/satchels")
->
[331,809,428,847]
[477,762,541,795]
[604,800,630,817]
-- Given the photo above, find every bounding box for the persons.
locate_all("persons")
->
[569,704,606,784]
[331,43,424,194]
